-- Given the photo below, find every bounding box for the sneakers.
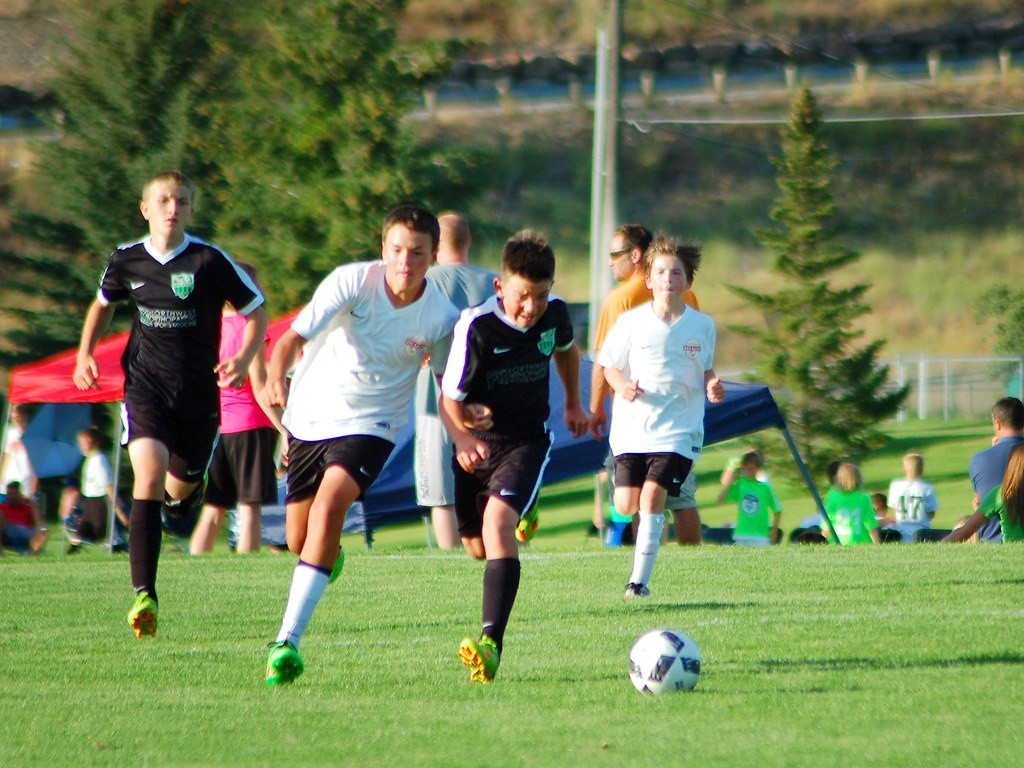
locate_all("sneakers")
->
[128,591,158,639]
[266,639,304,687]
[458,634,499,685]
[327,545,345,584]
[514,495,539,543]
[623,582,649,599]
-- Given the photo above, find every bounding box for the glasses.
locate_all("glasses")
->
[609,248,631,261]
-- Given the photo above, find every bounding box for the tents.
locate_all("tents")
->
[7,304,303,562]
[226,351,824,553]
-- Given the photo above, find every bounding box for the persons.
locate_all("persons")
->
[821,457,895,545]
[266,206,491,687]
[941,397,1024,542]
[189,261,279,553]
[411,212,505,553]
[881,454,937,543]
[71,170,266,634]
[584,224,708,548]
[597,236,722,599]
[0,402,49,561]
[63,426,207,557]
[438,227,591,684]
[707,441,781,545]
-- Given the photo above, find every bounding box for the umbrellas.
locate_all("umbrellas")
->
[18,404,94,479]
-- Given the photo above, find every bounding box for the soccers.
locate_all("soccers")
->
[628,629,701,696]
